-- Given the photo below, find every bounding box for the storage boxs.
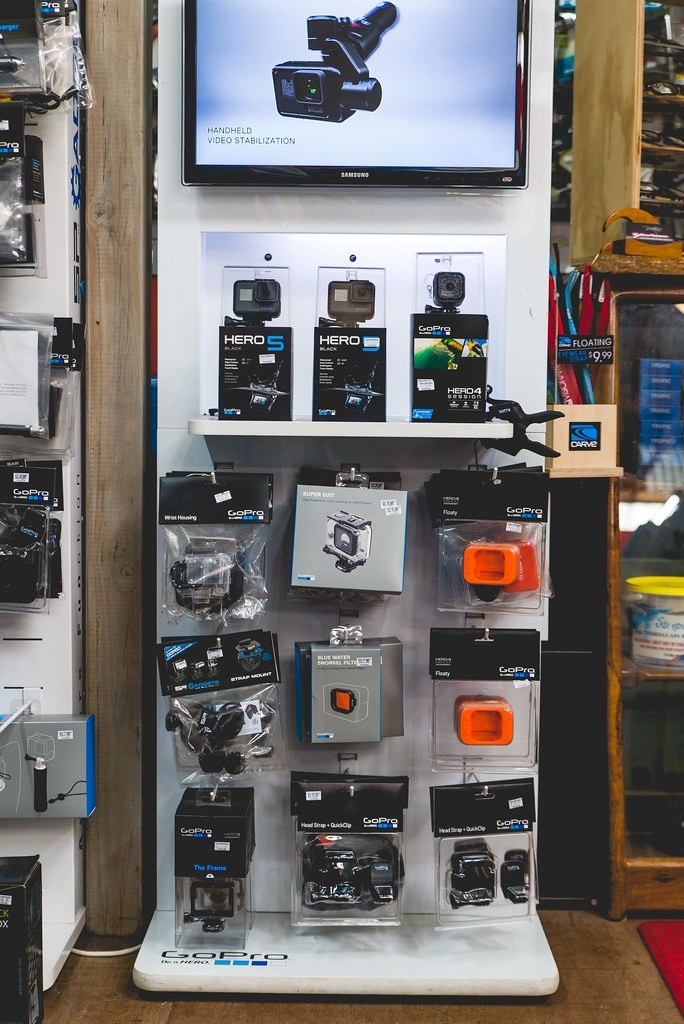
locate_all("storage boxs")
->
[296,639,405,744]
[216,326,295,420]
[410,312,490,422]
[0,711,97,818]
[311,328,387,424]
[547,403,617,468]
[0,854,45,1024]
[630,358,683,480]
[289,469,409,598]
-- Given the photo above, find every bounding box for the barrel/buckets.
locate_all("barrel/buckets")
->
[623,576,684,671]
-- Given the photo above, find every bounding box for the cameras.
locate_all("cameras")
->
[328,280,375,323]
[233,279,281,321]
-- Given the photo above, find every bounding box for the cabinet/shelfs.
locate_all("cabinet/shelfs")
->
[570,0,684,269]
[553,258,684,918]
[131,408,560,997]
[0,1,92,995]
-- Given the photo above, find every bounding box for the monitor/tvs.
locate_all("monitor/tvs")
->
[182,0,532,191]
